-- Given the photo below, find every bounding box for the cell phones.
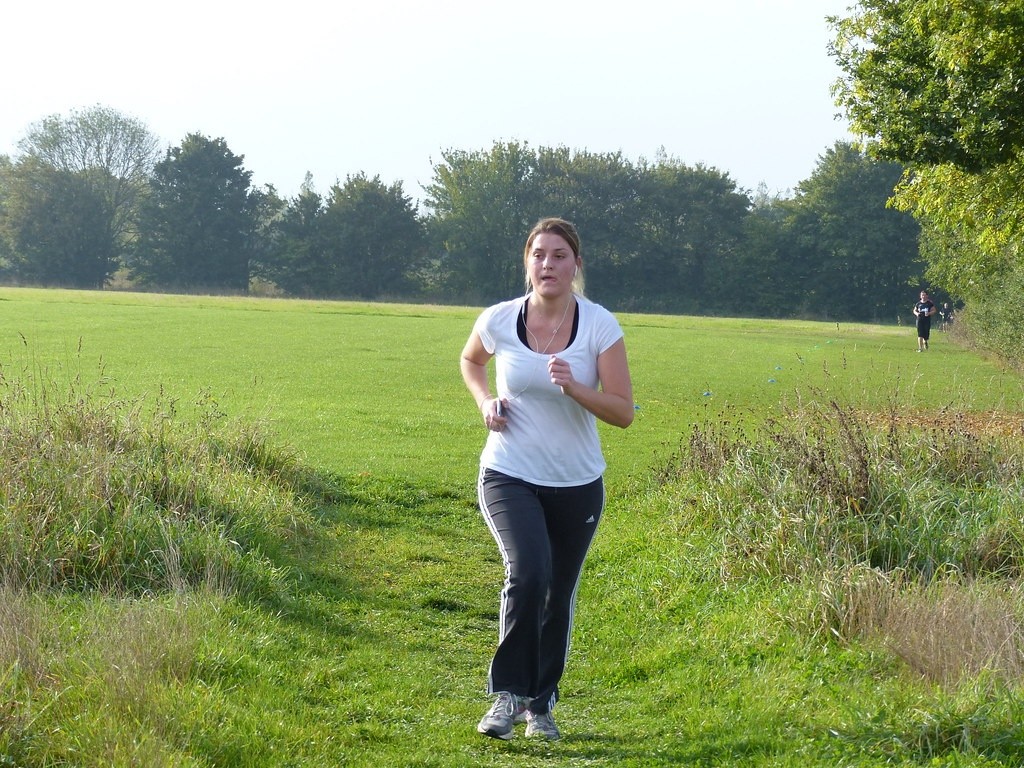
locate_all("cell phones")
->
[496,397,503,418]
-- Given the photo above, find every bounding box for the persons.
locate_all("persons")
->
[939,303,951,332]
[460,219,634,743]
[913,291,936,352]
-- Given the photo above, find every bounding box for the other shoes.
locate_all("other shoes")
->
[924,343,928,349]
[918,349,923,352]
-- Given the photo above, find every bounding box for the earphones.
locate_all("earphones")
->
[574,265,578,276]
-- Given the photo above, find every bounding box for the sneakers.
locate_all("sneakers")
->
[525,710,560,741]
[478,690,521,740]
